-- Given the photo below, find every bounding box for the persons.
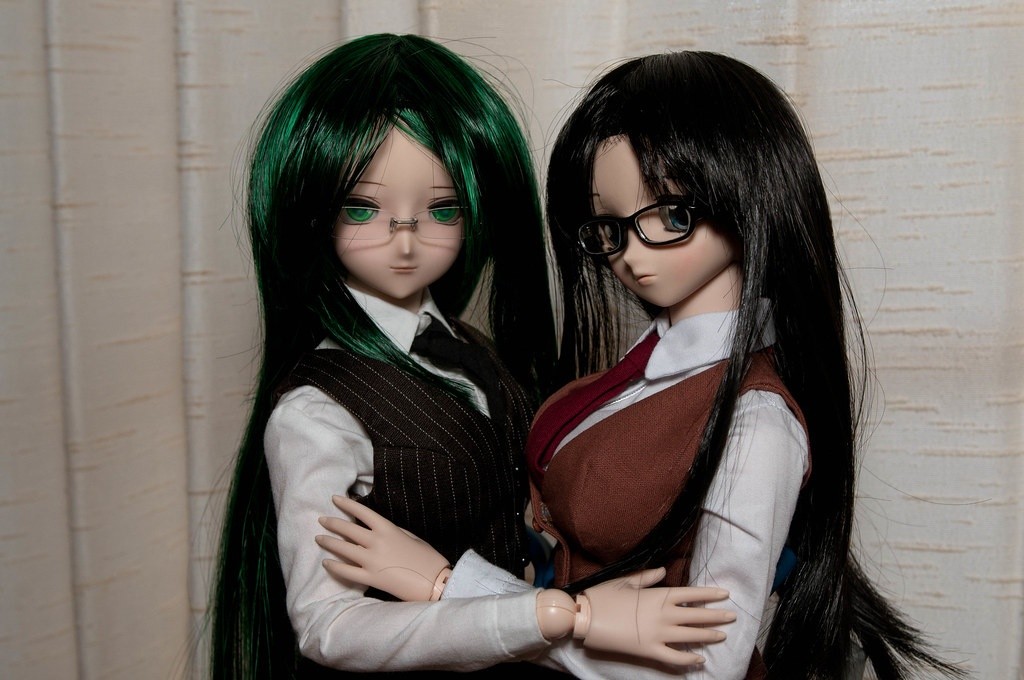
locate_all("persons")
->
[316,53,973,680]
[208,33,738,680]
